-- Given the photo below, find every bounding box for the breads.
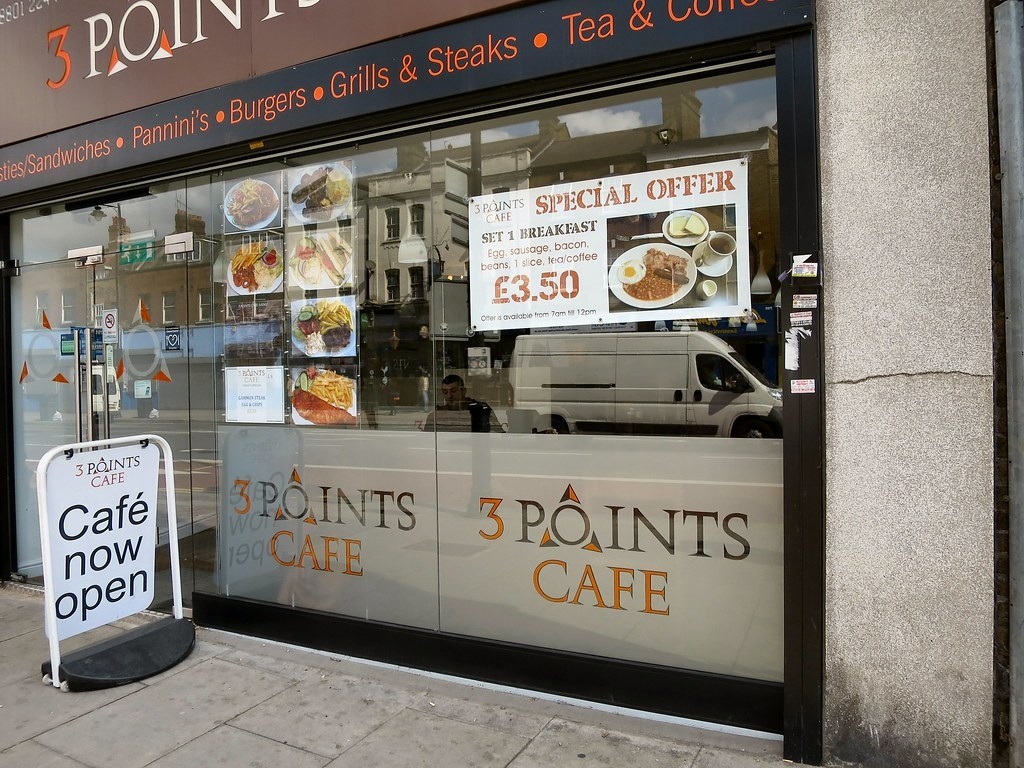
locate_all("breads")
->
[668,213,706,238]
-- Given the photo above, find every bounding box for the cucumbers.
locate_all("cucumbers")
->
[298,311,313,321]
[294,371,308,391]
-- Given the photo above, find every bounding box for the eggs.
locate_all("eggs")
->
[618,260,647,284]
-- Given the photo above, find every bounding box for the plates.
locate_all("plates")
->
[289,163,353,223]
[692,240,733,277]
[227,260,283,296]
[289,233,353,290]
[292,369,357,426]
[662,210,710,246]
[224,180,279,230]
[608,243,697,309]
[703,231,737,266]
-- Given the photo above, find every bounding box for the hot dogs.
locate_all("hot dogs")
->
[314,231,353,287]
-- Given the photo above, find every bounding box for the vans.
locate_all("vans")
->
[506,329,784,440]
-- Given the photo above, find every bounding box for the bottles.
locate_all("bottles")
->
[291,295,356,357]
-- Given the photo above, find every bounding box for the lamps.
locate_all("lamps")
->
[397,171,428,264]
[388,329,399,350]
[750,229,772,296]
[365,259,376,277]
[430,240,451,253]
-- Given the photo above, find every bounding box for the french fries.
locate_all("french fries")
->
[316,300,354,334]
[226,176,265,212]
[308,369,354,413]
[229,240,265,271]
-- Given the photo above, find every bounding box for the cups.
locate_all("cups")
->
[696,280,717,301]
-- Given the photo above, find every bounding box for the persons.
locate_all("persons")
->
[424,374,507,433]
[385,375,400,416]
[415,373,432,412]
[362,379,380,430]
